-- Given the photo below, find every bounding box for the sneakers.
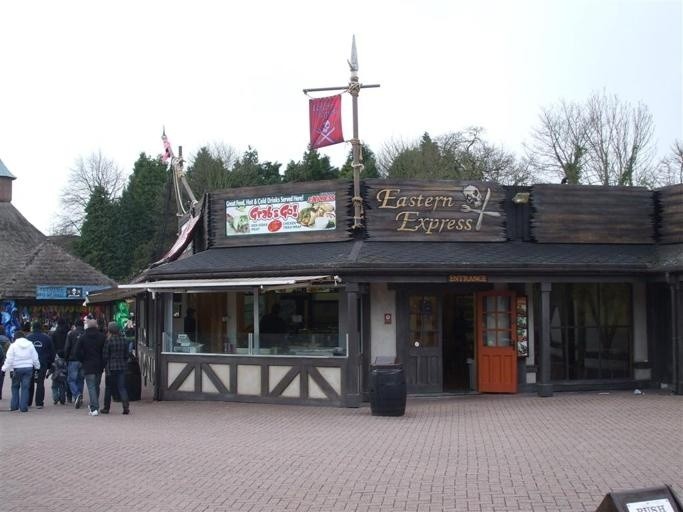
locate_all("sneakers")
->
[54,393,82,409]
[88,404,109,415]
[36,406,42,409]
[124,409,129,415]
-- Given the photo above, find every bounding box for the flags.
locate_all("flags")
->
[307,94,344,151]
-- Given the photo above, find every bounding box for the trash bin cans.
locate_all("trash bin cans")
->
[595,483,683,512]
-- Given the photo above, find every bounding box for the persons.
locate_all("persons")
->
[0,311,135,417]
[183,309,200,341]
[259,303,285,347]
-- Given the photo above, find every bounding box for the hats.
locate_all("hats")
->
[109,322,119,333]
